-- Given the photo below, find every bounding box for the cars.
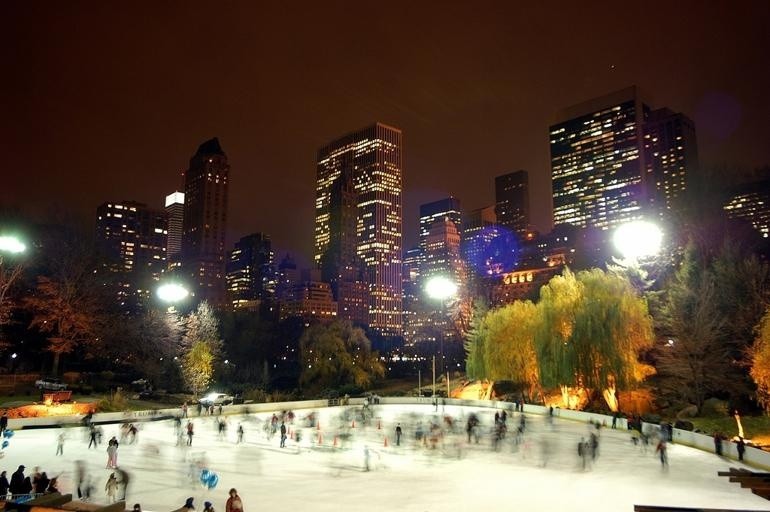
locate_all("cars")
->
[198,391,232,406]
[35,376,71,392]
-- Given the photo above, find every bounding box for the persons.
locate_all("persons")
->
[0,391,677,476]
[1,476,244,512]
[732,437,745,460]
[712,428,727,455]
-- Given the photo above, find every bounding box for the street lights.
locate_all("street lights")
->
[427,274,458,375]
